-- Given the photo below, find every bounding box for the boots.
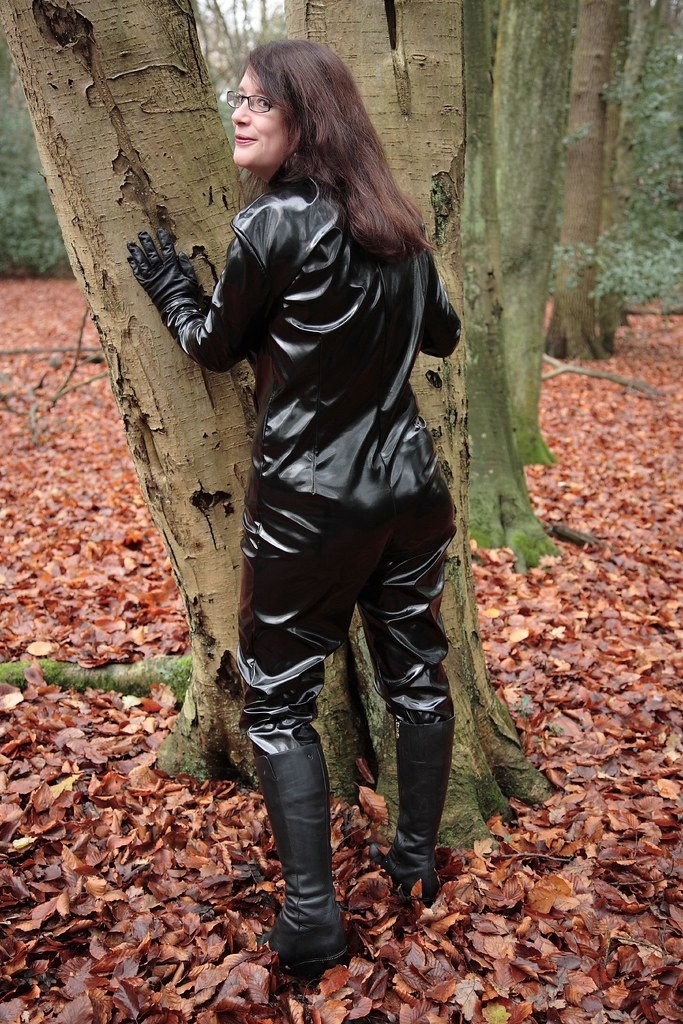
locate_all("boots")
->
[253,743,349,980]
[369,716,455,907]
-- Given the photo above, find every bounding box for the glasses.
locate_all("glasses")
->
[227,91,286,113]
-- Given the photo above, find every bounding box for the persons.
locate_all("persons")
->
[127,38,462,979]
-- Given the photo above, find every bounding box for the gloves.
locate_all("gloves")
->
[127,227,199,312]
